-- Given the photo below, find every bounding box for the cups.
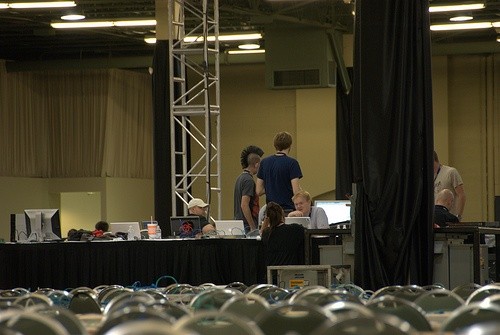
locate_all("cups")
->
[147,224,157,239]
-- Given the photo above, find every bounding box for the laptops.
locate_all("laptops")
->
[285,216,312,230]
[110,222,143,240]
[170,216,201,238]
[214,220,246,237]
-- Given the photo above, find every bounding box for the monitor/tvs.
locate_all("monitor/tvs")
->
[314,199,351,225]
[24,208,62,241]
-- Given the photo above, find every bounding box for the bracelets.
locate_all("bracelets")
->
[456,215,462,220]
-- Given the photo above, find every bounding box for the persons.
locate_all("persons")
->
[433,151,465,221]
[260,202,306,266]
[93,221,109,237]
[256,132,303,216]
[288,191,329,229]
[187,199,214,233]
[435,189,459,223]
[233,146,264,234]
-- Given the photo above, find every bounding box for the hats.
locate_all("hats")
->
[188,198,209,209]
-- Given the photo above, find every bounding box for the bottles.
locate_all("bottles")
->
[127,226,135,240]
[156,226,161,239]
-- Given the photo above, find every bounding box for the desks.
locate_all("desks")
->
[0,236,261,285]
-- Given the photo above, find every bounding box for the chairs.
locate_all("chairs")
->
[0,282,500,335]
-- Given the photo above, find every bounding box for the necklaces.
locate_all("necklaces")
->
[277,152,285,155]
[244,170,250,173]
